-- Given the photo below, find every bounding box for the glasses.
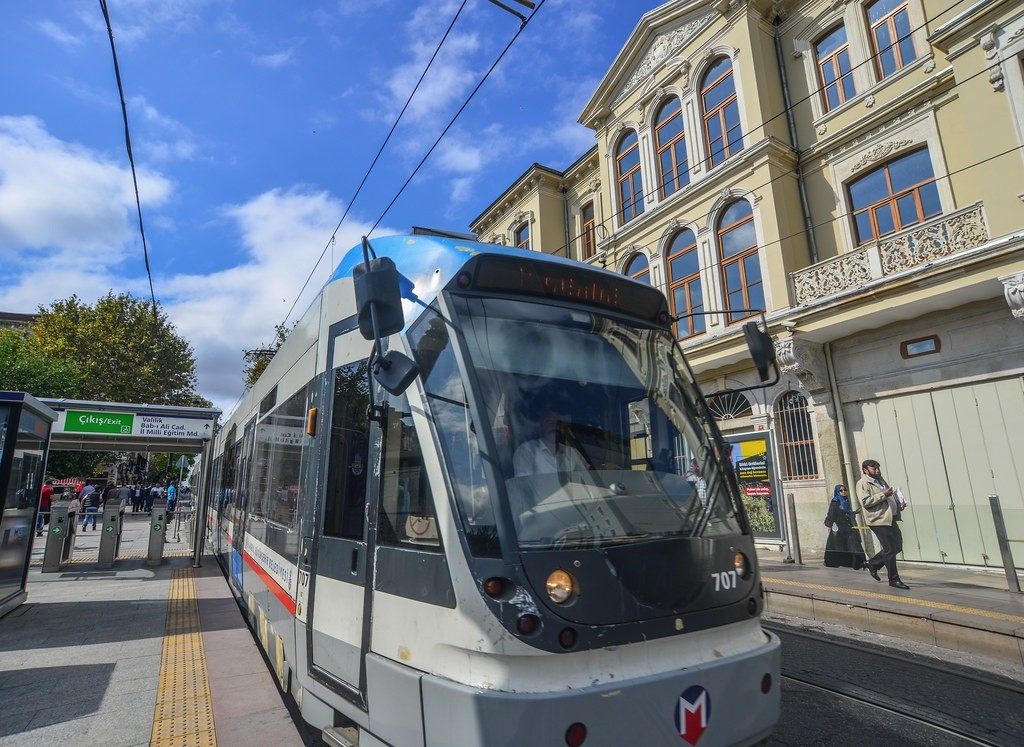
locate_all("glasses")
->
[840,488,848,492]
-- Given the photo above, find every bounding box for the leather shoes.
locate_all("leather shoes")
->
[889,579,910,589]
[863,561,881,581]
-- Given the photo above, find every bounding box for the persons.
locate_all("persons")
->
[683,458,707,509]
[36,479,55,536]
[167,481,176,518]
[513,410,619,543]
[180,485,193,495]
[824,484,867,570]
[64,492,80,535]
[81,484,101,532]
[856,460,909,589]
[73,481,165,517]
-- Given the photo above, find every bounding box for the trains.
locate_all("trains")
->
[186,224,785,747]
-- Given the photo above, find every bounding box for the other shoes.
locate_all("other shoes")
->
[82,525,86,531]
[91,526,96,530]
